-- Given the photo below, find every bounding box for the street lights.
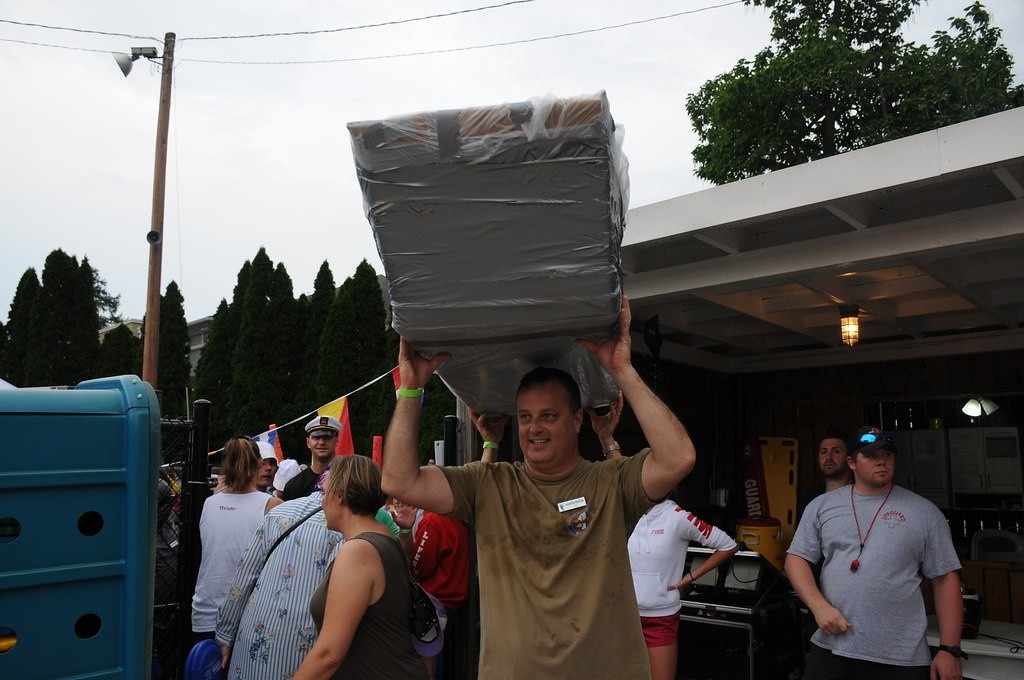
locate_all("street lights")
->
[114,31,178,391]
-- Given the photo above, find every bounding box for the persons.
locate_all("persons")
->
[381,294,695,680]
[375,498,470,680]
[282,417,342,503]
[153,469,183,680]
[255,440,308,498]
[813,431,854,498]
[191,435,285,641]
[292,456,431,680]
[468,390,624,462]
[784,427,968,680]
[626,499,740,680]
[215,464,346,680]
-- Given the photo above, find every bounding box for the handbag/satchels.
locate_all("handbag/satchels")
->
[409,586,438,637]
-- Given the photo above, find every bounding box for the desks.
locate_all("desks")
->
[924,614,1024,680]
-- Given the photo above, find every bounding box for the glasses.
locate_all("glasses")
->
[850,433,894,453]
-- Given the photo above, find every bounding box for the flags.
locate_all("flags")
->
[392,367,424,407]
[254,431,284,469]
[318,397,354,457]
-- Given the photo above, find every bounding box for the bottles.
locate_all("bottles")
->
[959,581,976,610]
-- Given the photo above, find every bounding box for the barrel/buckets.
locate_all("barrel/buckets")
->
[736,516,783,572]
[962,609,983,638]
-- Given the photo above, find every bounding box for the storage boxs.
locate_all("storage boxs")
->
[685,547,719,589]
[722,546,765,594]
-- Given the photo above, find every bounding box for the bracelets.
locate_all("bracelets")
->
[398,389,423,398]
[483,441,499,450]
[603,442,621,454]
[399,529,412,534]
[690,572,697,582]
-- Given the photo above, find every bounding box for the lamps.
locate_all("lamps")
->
[837,300,861,346]
[960,395,1001,425]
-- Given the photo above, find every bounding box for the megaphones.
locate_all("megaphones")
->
[147,231,161,245]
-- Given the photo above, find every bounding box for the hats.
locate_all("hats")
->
[255,440,278,465]
[305,415,342,437]
[847,425,892,457]
[405,581,448,657]
[272,458,302,491]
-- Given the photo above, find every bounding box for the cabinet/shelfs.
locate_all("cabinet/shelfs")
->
[886,428,952,510]
[946,426,1024,512]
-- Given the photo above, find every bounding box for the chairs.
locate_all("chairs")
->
[971,528,1024,563]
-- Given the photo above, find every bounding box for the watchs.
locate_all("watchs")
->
[939,645,968,660]
